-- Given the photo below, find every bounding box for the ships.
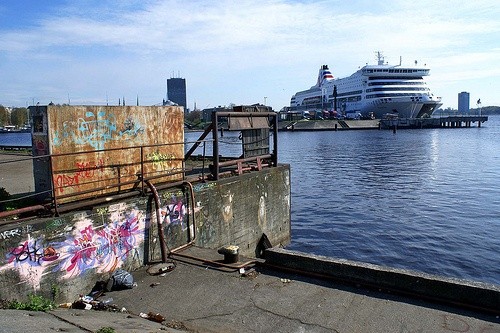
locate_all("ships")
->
[289,50,443,127]
